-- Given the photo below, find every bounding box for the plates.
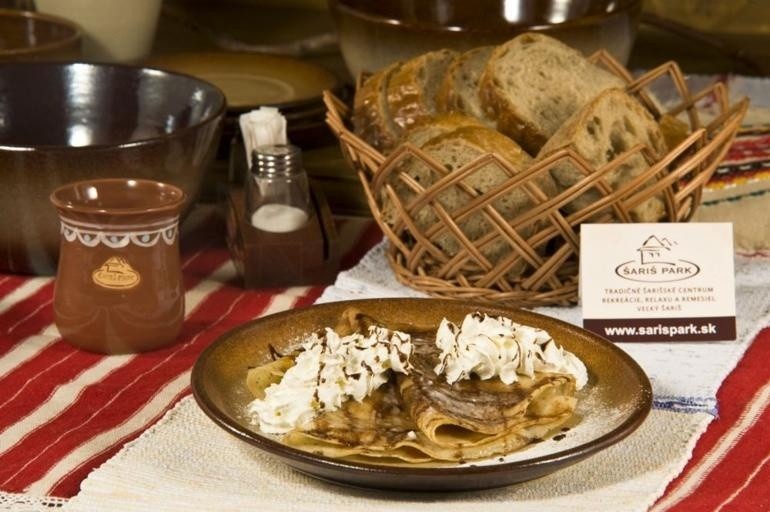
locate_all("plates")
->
[611,71,768,205]
[187,299,657,492]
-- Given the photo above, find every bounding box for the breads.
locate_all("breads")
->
[354,30,689,281]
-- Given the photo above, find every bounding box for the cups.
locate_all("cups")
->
[46,177,185,351]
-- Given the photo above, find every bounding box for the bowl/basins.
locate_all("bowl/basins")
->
[157,55,349,145]
[2,60,230,274]
[327,1,647,84]
[1,9,80,61]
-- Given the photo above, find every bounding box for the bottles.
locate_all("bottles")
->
[245,142,314,233]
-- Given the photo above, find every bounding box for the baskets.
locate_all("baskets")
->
[324,46,750,305]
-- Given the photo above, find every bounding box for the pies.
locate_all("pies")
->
[245,306,589,465]
